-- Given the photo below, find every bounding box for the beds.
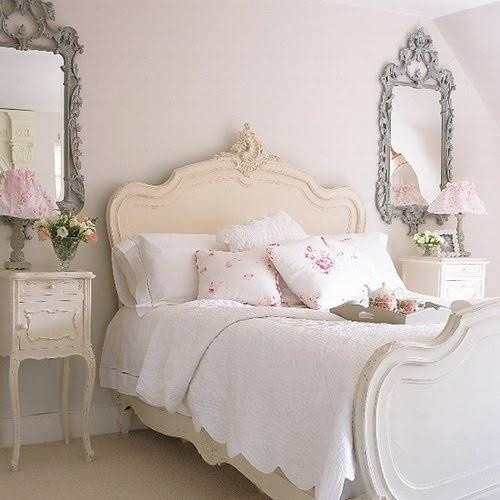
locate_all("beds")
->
[104,123,500,500]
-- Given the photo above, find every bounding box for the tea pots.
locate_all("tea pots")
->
[362,281,403,314]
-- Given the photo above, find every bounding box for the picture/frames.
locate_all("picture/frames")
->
[437,231,461,257]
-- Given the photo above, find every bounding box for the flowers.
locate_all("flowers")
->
[37,213,98,243]
[412,231,444,244]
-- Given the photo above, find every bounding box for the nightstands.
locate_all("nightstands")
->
[0,271,96,472]
[399,257,491,300]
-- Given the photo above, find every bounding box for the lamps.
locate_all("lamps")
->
[0,168,53,269]
[427,181,487,256]
[390,185,429,205]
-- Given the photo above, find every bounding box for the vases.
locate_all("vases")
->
[49,238,84,271]
[418,246,441,257]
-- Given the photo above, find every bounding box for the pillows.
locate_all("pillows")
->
[111,212,404,309]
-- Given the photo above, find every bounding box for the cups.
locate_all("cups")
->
[397,296,426,314]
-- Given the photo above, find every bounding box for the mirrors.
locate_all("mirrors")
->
[0,0,86,226]
[375,27,456,236]
